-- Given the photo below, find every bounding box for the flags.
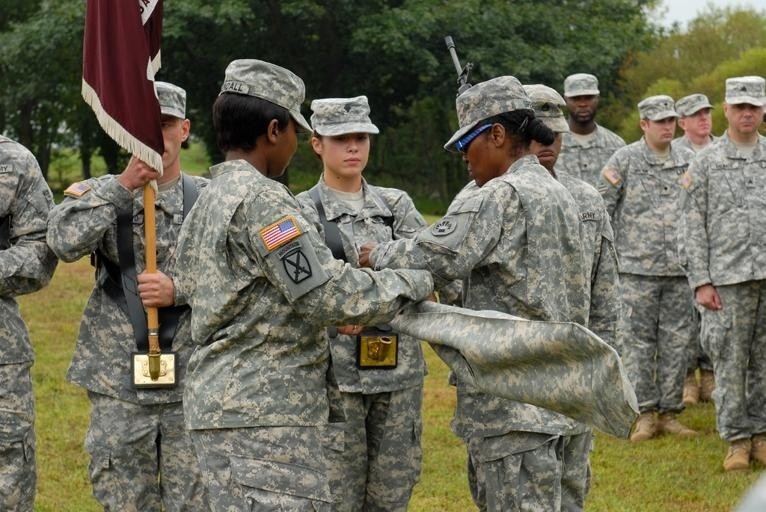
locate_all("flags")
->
[81,1,165,198]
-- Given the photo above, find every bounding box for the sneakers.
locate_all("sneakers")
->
[657,411,700,436]
[700,370,717,400]
[722,438,752,471]
[751,435,766,465]
[682,367,701,405]
[630,410,657,441]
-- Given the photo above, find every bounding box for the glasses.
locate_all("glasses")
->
[454,123,492,152]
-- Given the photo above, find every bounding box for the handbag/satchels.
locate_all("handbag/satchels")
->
[390,301,640,438]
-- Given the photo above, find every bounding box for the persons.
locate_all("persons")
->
[175,58,437,512]
[359,73,592,512]
[557,73,626,188]
[443,85,619,382]
[681,75,765,468]
[0,135,57,511]
[597,95,701,440]
[294,97,460,511]
[46,83,210,512]
[671,93,730,405]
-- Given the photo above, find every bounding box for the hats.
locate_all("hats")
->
[725,76,766,107]
[218,59,315,135]
[310,95,380,137]
[522,83,571,132]
[637,95,680,121]
[674,93,715,118]
[563,73,601,97]
[444,75,535,154]
[155,80,186,120]
